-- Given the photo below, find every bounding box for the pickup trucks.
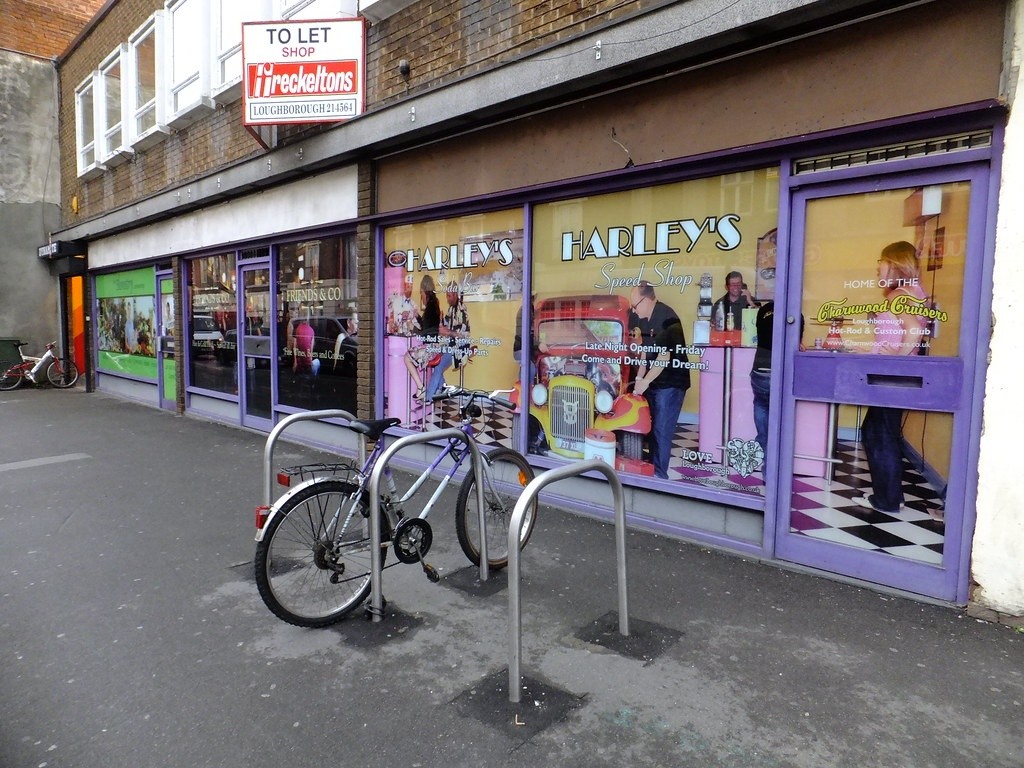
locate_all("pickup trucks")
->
[224,315,358,376]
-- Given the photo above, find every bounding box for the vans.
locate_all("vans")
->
[191,312,224,359]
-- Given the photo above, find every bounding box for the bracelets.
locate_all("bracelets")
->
[635,376,643,382]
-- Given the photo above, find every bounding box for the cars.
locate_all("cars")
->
[507,291,653,458]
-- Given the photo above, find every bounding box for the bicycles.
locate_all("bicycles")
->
[0,341,79,391]
[253,382,538,629]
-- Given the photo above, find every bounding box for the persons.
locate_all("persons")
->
[852,241,928,512]
[165,302,174,336]
[389,274,419,335]
[125,302,135,355]
[711,271,761,331]
[291,309,320,389]
[331,307,359,374]
[404,276,440,400]
[137,333,151,355]
[628,309,647,395]
[748,301,806,484]
[629,278,691,481]
[514,291,537,384]
[416,281,471,405]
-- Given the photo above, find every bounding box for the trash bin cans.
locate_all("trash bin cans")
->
[1,336,23,388]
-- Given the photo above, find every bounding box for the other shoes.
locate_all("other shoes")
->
[863,492,905,509]
[851,493,880,510]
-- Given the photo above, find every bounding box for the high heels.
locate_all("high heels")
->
[411,385,424,399]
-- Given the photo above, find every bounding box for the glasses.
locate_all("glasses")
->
[878,259,886,264]
[632,297,645,310]
[728,284,741,288]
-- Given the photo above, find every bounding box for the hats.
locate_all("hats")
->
[405,274,415,284]
